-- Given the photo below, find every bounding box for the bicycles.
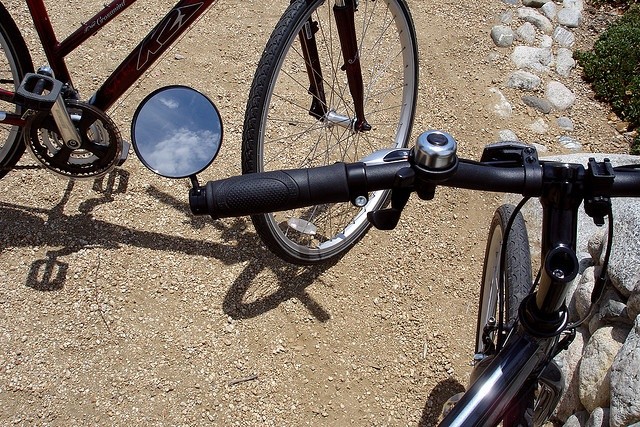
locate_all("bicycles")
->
[130,84,639,425]
[0,0,418,265]
